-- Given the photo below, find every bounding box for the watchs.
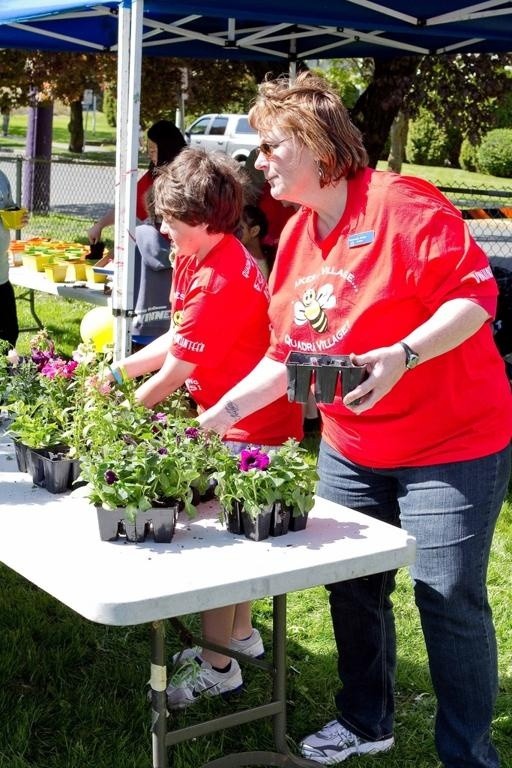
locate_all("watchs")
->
[398,338,420,371]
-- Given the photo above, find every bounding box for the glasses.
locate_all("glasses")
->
[256,138,293,156]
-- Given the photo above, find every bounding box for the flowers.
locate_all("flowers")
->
[1,328,317,516]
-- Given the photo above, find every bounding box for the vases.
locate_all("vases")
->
[284,350,366,405]
[0,205,29,230]
[227,494,308,542]
[93,498,180,543]
[10,236,108,283]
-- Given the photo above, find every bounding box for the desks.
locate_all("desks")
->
[8,251,114,333]
[0,412,416,768]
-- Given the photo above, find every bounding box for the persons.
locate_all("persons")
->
[1,169,31,355]
[88,119,187,244]
[189,68,512,766]
[88,150,304,715]
[238,203,272,283]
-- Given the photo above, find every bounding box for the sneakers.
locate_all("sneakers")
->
[147,654,243,709]
[298,720,394,765]
[173,629,264,670]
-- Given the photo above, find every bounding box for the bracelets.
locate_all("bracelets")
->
[109,362,128,384]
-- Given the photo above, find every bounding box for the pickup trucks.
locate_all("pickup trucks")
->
[183,112,261,167]
